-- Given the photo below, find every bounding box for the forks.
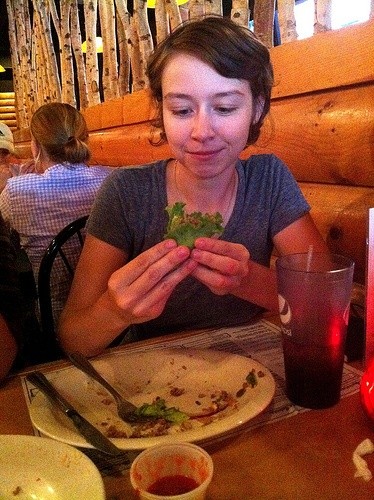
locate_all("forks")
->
[70,350,156,422]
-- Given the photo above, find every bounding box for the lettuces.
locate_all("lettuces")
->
[164,202,224,250]
[132,397,191,423]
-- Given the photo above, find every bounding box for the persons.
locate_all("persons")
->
[0,122,14,162]
[58,13,331,360]
[0,211,38,382]
[0,99,136,348]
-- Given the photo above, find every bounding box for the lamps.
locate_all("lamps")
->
[81,21,102,54]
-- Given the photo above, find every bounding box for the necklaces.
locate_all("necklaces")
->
[174,163,235,225]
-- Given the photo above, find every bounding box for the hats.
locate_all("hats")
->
[0,123,14,153]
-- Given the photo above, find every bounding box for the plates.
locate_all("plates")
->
[29,349,274,450]
[0,434,106,499]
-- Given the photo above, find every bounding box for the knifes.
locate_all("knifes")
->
[27,370,123,455]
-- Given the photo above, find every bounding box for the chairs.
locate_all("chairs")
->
[38,216,89,331]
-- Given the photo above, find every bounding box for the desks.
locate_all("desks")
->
[0,312,374,500]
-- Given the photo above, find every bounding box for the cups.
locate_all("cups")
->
[276,253,355,407]
[130,442,213,500]
